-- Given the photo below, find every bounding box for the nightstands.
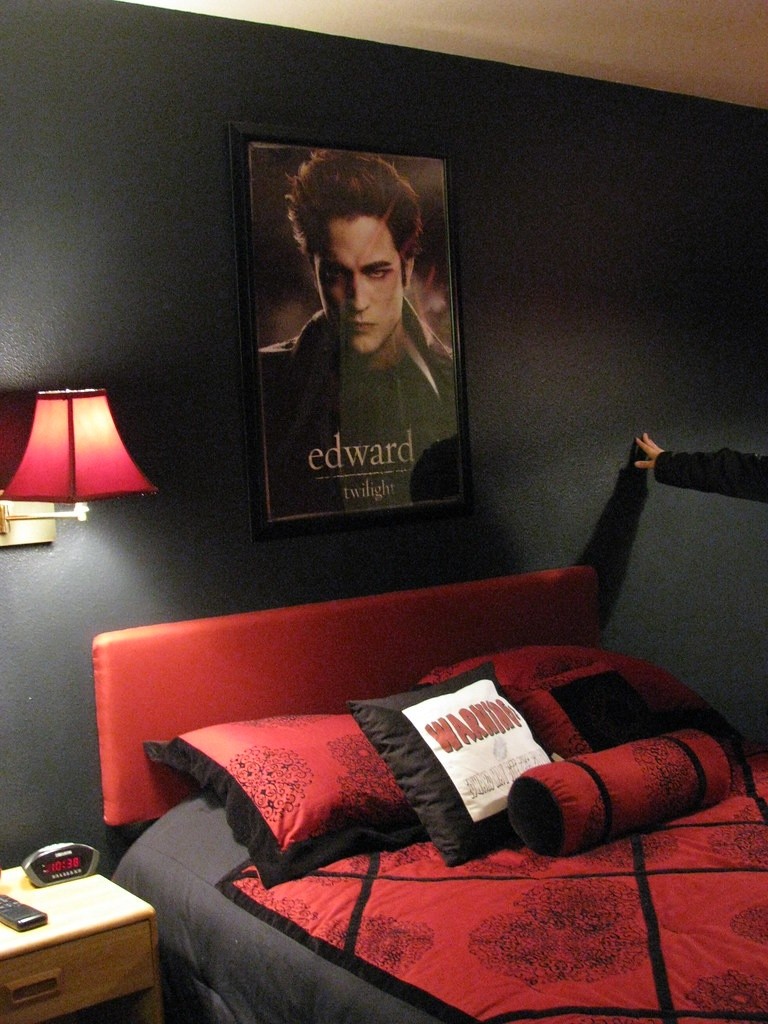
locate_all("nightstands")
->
[0,866,165,1023]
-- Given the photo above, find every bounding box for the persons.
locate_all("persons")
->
[635,433,768,503]
[258,148,461,515]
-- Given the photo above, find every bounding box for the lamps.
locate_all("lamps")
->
[0,385,158,549]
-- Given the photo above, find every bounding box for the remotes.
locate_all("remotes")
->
[0,895,48,932]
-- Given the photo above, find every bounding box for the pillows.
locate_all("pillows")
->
[347,660,557,867]
[503,654,660,761]
[144,713,431,894]
[507,728,731,857]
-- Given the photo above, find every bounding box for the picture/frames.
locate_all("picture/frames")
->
[226,122,479,542]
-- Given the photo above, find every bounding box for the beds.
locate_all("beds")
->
[92,564,768,1023]
